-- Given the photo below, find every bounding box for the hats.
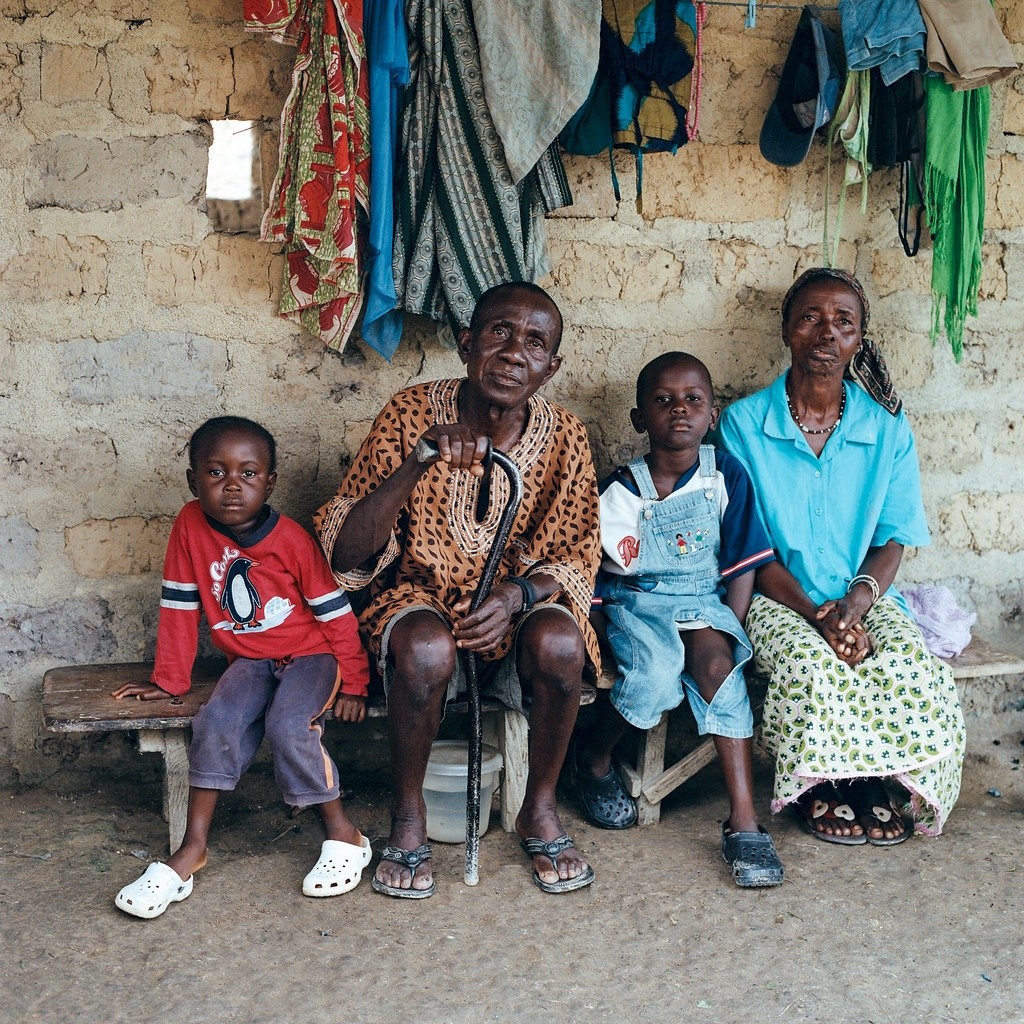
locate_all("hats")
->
[759,6,843,167]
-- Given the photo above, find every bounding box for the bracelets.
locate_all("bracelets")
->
[509,575,536,613]
[848,575,879,603]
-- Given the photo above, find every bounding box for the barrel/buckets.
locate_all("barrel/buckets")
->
[422,739,504,843]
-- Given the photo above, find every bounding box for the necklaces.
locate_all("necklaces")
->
[786,382,845,433]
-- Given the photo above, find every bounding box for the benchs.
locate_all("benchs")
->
[38,631,1023,887]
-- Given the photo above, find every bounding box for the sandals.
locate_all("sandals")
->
[570,740,640,830]
[721,820,783,888]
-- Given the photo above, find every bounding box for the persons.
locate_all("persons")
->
[707,266,968,847]
[113,416,371,920]
[314,282,603,899]
[568,352,784,885]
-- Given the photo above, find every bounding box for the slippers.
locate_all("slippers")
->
[114,860,194,919]
[844,784,910,845]
[302,836,372,896]
[521,833,594,893]
[799,791,867,845]
[372,841,434,899]
